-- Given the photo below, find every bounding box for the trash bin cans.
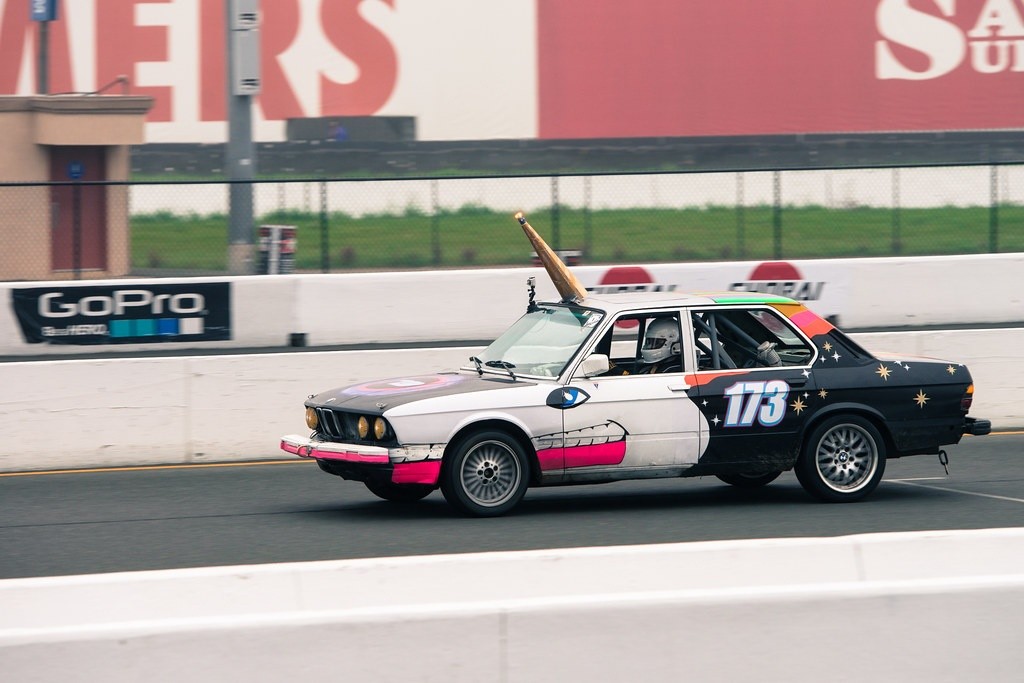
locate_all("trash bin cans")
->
[531,249,583,267]
[258,225,298,275]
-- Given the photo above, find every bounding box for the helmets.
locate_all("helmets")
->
[641,318,681,363]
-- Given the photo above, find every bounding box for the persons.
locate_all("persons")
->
[602,317,684,375]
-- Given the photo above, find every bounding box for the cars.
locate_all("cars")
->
[277,209,993,517]
